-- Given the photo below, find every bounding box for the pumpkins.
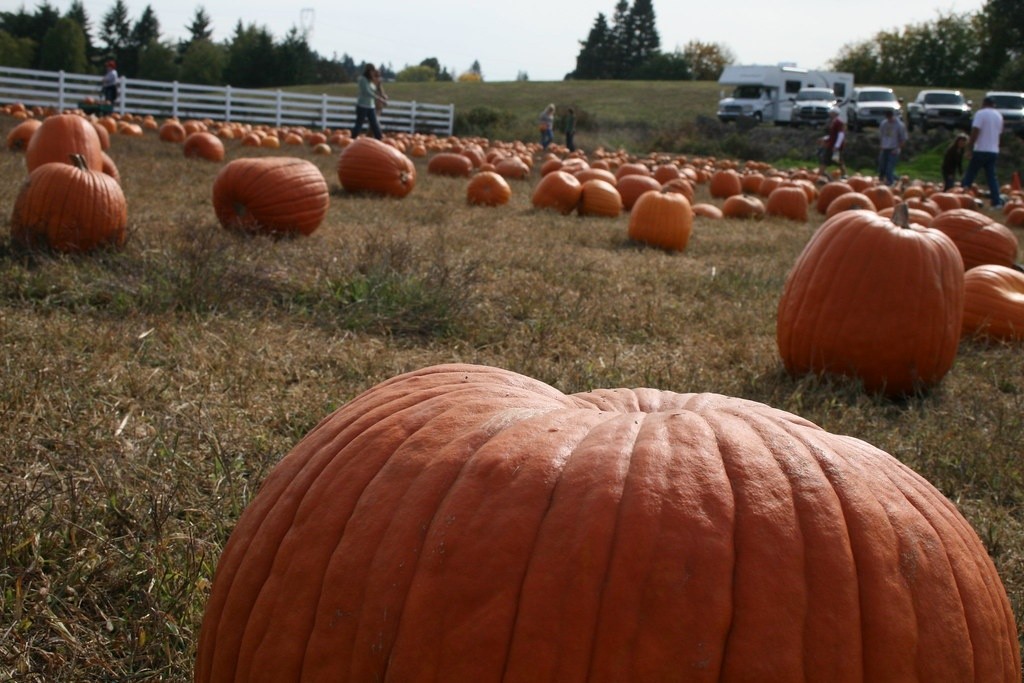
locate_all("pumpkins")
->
[0,100,1024,392]
[196,364,1016,683]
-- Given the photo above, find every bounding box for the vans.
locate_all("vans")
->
[717,64,855,122]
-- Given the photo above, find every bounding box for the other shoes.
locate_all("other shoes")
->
[993,198,1007,208]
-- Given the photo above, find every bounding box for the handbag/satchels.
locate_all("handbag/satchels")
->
[539,123,547,132]
[816,146,833,166]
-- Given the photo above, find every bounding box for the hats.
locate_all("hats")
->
[827,106,841,115]
[105,60,115,69]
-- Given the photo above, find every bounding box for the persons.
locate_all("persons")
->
[539,103,556,149]
[564,108,578,152]
[817,106,848,181]
[101,61,118,115]
[879,112,907,186]
[941,133,968,191]
[961,97,1003,212]
[352,63,389,141]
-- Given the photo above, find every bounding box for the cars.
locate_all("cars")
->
[789,86,843,127]
[982,91,1024,125]
[847,86,904,132]
[907,90,973,135]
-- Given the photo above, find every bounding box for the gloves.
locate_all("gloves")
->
[570,127,576,136]
